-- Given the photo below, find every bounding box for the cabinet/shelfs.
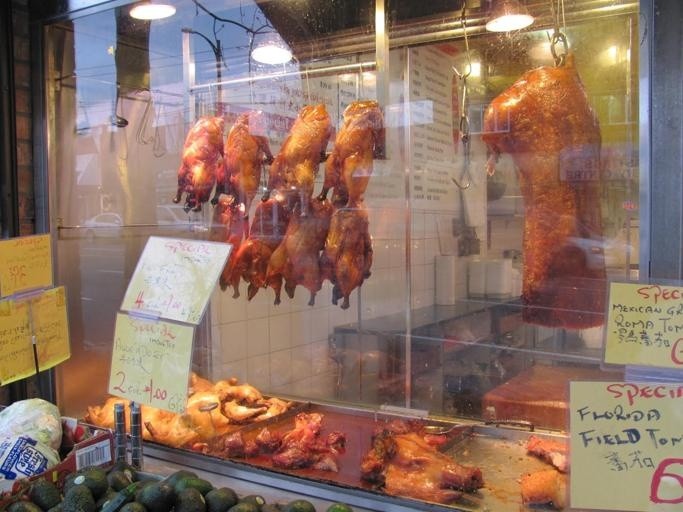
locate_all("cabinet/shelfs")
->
[458,291,580,382]
[333,297,501,419]
[467,87,639,135]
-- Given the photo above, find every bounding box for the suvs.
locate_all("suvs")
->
[152,203,225,237]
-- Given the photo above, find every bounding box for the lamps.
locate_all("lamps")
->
[250,29,294,65]
[486,0,535,33]
[129,0,176,19]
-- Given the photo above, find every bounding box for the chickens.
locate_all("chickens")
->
[85,370,291,449]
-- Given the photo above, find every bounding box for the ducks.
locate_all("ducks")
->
[237,193,290,306]
[312,199,376,309]
[320,101,385,209]
[258,198,328,308]
[225,110,271,209]
[175,111,224,209]
[264,101,331,219]
[205,205,250,301]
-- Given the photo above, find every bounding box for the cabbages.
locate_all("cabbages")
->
[0,397,63,492]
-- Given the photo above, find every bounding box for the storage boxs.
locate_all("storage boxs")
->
[488,195,516,216]
[433,255,523,305]
[0,416,116,511]
[515,196,525,214]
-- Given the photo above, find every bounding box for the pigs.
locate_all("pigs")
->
[360,431,485,505]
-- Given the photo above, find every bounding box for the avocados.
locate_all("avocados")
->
[7,461,353,512]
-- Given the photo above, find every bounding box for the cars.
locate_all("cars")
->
[76,210,126,241]
[547,209,639,275]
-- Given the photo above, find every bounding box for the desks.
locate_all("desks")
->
[481,360,624,431]
